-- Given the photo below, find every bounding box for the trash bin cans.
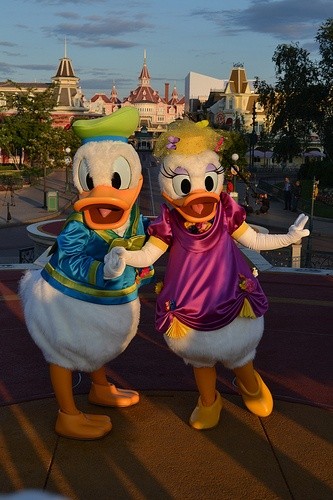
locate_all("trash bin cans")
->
[47,191,58,212]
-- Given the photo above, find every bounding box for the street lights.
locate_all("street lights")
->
[233,154,238,194]
[305,176,320,258]
[64,148,71,194]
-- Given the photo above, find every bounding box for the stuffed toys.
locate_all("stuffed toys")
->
[19,105,154,439]
[104,117,311,430]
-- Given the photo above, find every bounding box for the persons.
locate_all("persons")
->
[256,194,270,214]
[283,178,291,211]
[291,180,302,212]
